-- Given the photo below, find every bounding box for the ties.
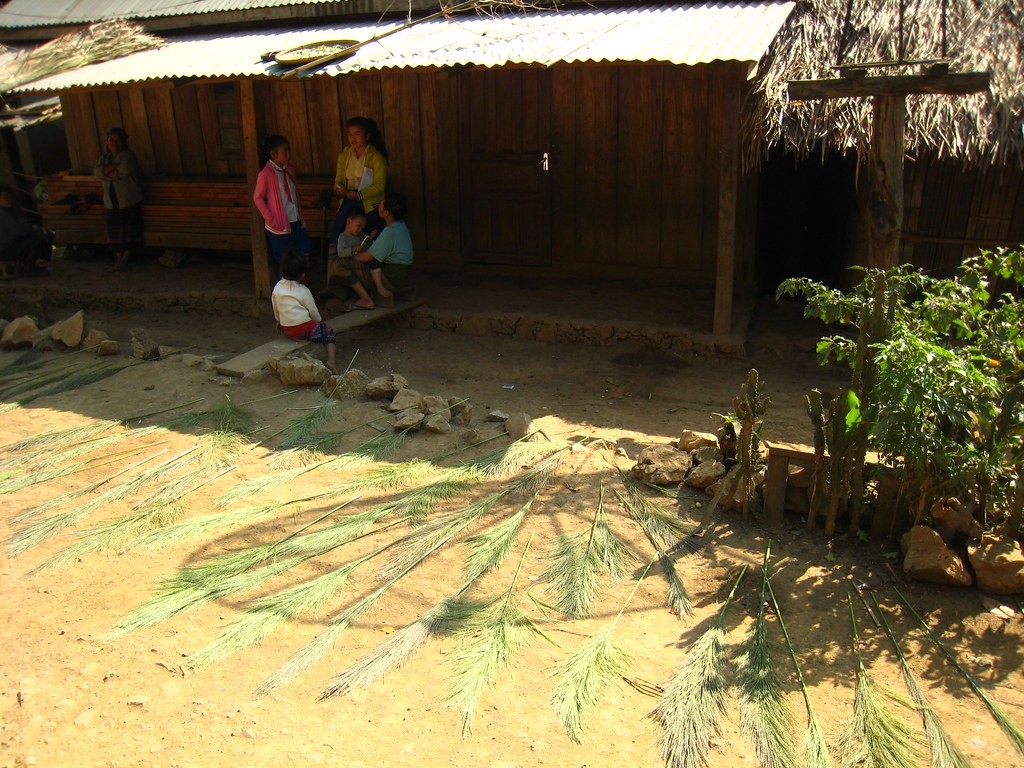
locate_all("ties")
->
[271,164,297,203]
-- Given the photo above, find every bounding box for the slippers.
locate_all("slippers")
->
[347,303,376,311]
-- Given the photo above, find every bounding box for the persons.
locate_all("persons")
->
[252,134,315,292]
[94,126,144,272]
[271,252,338,376]
[317,115,414,311]
[0,186,57,277]
